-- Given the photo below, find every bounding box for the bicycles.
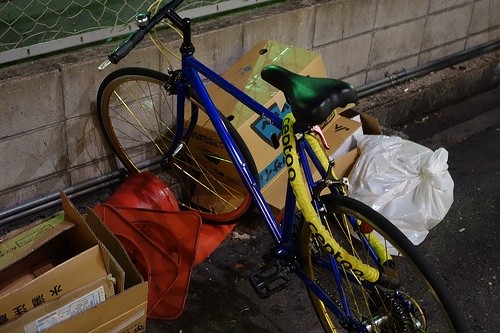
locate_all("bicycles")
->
[96,1,469,333]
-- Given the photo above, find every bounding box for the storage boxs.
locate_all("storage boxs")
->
[0,205,150,333]
[184,37,363,213]
[0,189,126,333]
